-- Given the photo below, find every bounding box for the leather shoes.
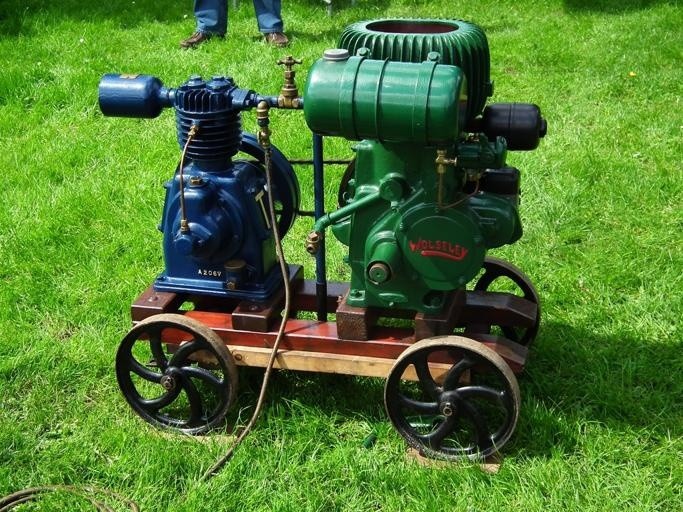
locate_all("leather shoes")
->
[266,32,289,46]
[180,31,225,48]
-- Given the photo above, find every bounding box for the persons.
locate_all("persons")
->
[177,1,288,49]
